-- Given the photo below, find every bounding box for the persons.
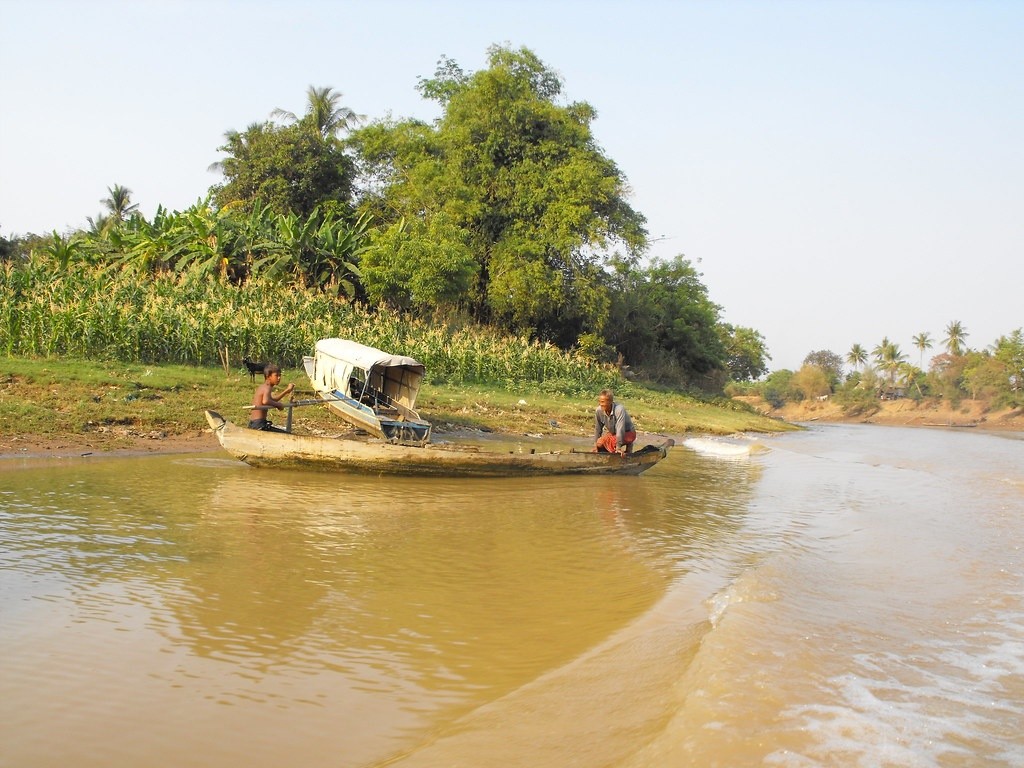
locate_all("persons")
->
[593,389,636,460]
[247,364,296,435]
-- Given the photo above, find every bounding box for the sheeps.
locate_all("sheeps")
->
[241,355,274,383]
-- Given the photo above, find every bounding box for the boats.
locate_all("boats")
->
[205,409,677,477]
[302,337,433,449]
